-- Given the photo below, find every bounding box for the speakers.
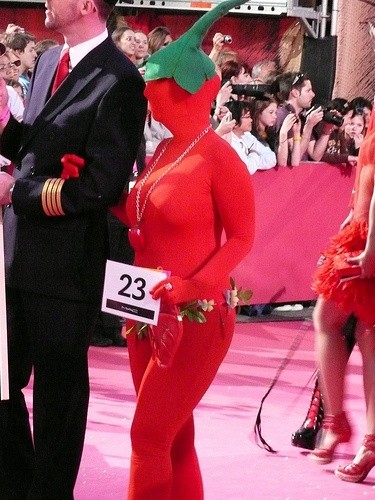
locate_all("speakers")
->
[299,35,337,108]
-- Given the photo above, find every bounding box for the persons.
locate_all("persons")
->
[0,1,148,500]
[292,100,375,483]
[1,23,372,171]
[61,41,257,500]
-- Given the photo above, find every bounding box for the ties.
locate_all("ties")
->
[51,48,70,96]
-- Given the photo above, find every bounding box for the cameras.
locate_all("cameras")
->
[222,79,274,100]
[314,103,344,126]
[222,35,232,44]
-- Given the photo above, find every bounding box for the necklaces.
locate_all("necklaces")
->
[128,124,213,252]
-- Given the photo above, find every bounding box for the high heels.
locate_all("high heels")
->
[307,412,352,464]
[335,434,375,481]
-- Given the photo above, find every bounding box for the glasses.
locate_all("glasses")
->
[292,72,305,85]
[356,108,364,115]
[0,64,15,73]
[14,60,21,67]
[242,115,251,118]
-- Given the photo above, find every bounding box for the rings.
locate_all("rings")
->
[164,282,173,291]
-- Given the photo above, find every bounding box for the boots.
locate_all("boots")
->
[292,315,361,449]
